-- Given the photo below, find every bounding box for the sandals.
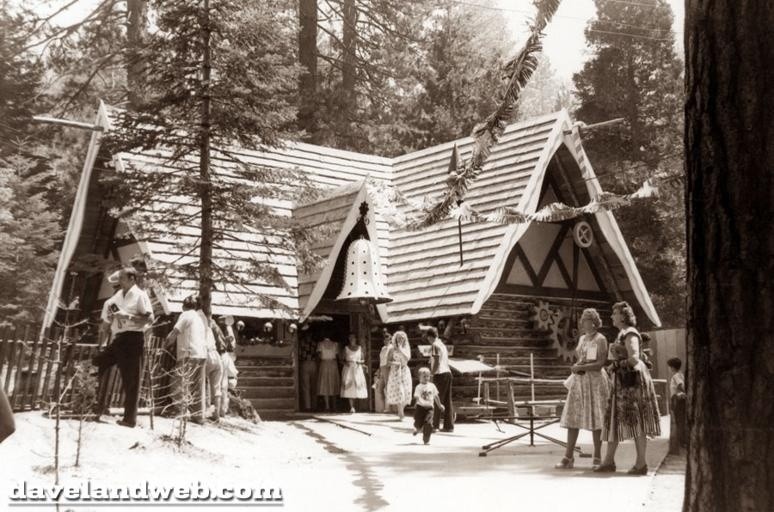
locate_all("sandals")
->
[349,406,356,413]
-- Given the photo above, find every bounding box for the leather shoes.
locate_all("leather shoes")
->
[116,419,137,428]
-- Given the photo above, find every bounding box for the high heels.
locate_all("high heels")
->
[592,462,617,473]
[554,454,575,469]
[625,463,649,476]
[592,456,602,471]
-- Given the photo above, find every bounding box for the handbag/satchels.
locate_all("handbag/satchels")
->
[615,357,644,389]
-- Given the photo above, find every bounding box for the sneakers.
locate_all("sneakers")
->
[189,410,231,425]
[412,429,420,436]
[423,442,432,445]
[430,427,454,433]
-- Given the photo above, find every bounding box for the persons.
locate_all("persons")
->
[90,266,238,428]
[590,300,663,475]
[411,365,445,446]
[373,331,391,413]
[312,331,344,412]
[667,356,687,398]
[382,329,414,420]
[425,326,454,434]
[552,305,609,472]
[297,331,320,413]
[340,332,370,413]
[639,329,656,370]
